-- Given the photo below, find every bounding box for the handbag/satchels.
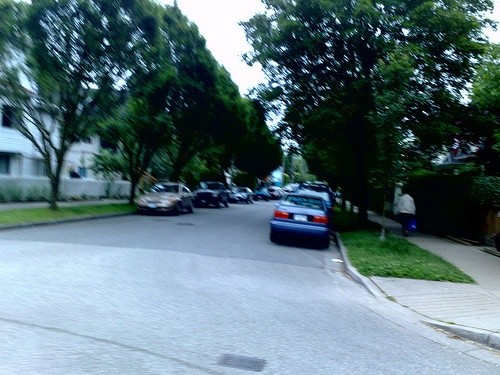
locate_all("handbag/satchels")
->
[407,218,418,233]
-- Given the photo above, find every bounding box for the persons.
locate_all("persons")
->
[393,188,417,237]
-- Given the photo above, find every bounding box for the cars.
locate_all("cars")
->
[130,181,335,250]
